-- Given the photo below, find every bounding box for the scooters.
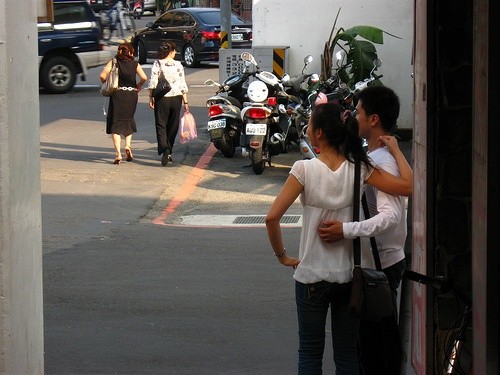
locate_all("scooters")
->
[126,0,143,20]
[204,52,383,174]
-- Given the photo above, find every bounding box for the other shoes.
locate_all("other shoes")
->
[161,150,174,167]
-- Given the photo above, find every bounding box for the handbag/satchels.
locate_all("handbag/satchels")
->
[180,111,197,144]
[152,60,172,103]
[101,58,119,97]
[349,265,394,322]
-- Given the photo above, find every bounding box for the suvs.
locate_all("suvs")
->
[37,0,104,94]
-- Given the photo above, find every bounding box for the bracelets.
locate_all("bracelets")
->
[149,95,153,97]
[184,102,188,104]
[274,248,286,256]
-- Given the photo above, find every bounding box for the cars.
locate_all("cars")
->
[130,7,252,67]
[143,0,157,15]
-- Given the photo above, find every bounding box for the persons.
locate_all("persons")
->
[265,103,414,375]
[318,85,408,319]
[99,44,147,165]
[148,41,189,166]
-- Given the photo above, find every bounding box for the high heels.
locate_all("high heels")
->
[124,146,133,162]
[113,152,123,164]
